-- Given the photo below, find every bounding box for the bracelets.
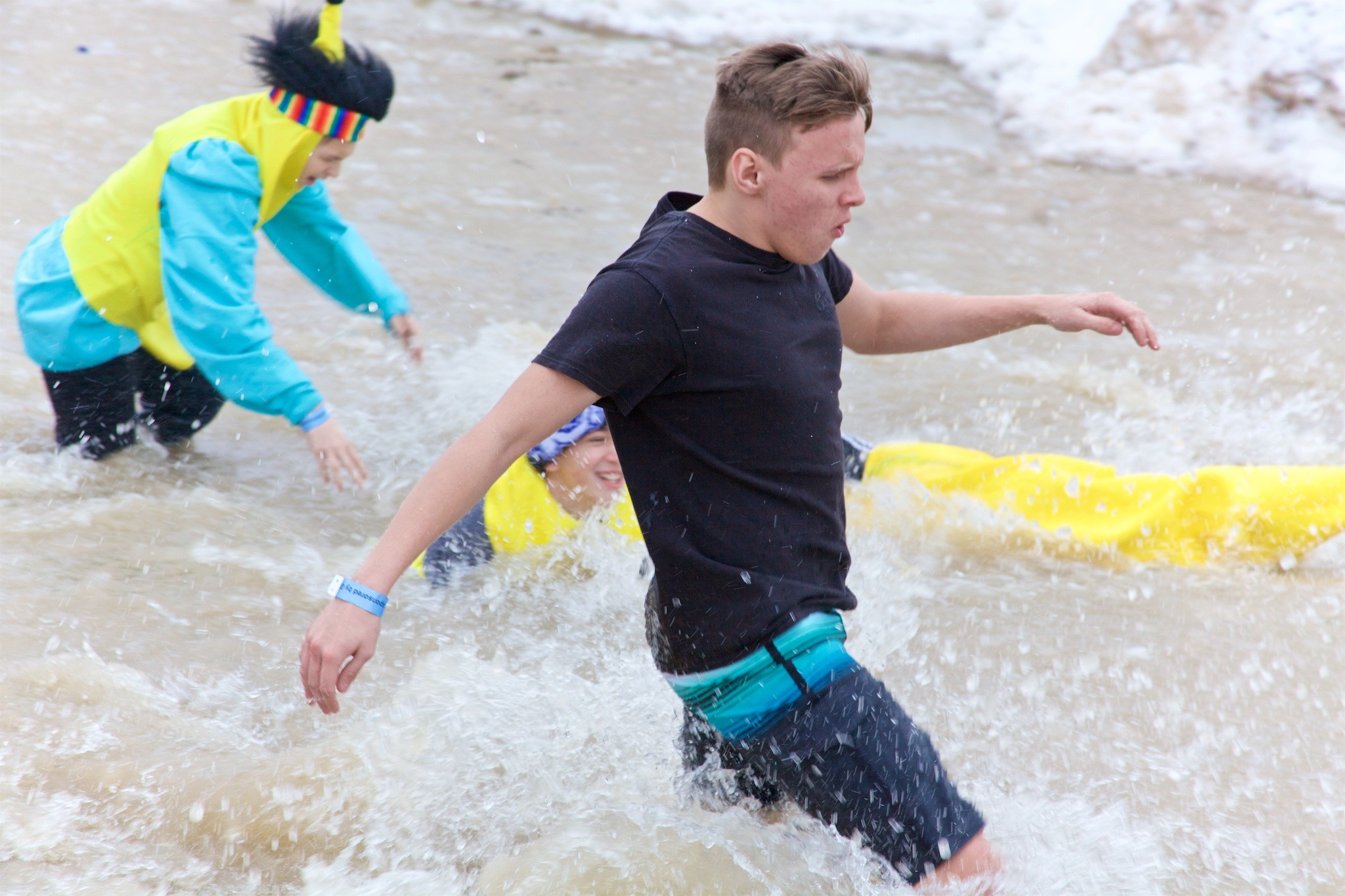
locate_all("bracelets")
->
[301,404,333,432]
[326,575,389,616]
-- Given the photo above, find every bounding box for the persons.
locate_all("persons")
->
[299,41,1160,890]
[412,402,645,580]
[15,0,422,494]
